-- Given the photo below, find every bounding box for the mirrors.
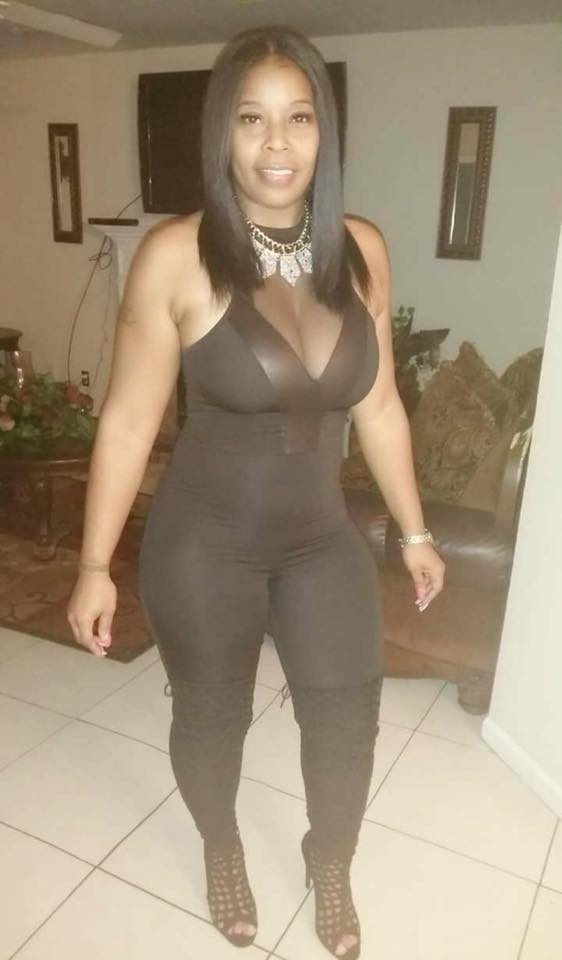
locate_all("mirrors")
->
[48,122,83,244]
[436,104,498,261]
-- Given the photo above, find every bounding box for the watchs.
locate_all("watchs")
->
[398,528,435,548]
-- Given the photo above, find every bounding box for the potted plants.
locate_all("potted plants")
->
[390,303,448,413]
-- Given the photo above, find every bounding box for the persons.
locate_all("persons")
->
[70,23,447,960]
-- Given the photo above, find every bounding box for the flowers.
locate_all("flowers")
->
[0,370,93,450]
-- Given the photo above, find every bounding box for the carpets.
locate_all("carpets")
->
[0,477,154,663]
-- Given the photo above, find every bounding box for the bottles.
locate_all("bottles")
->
[78,371,94,411]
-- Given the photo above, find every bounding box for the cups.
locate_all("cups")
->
[6,351,35,389]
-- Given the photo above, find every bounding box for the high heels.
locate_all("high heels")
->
[203,827,258,949]
[301,830,361,960]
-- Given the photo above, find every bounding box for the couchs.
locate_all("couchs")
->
[338,347,544,715]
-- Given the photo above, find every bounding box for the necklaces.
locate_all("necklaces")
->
[231,196,317,287]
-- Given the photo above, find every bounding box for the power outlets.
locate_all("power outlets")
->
[81,370,90,386]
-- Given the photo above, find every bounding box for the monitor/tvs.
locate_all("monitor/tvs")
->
[139,61,347,214]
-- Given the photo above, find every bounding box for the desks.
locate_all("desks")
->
[0,447,92,560]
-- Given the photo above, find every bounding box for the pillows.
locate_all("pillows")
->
[452,341,514,428]
[410,361,499,506]
